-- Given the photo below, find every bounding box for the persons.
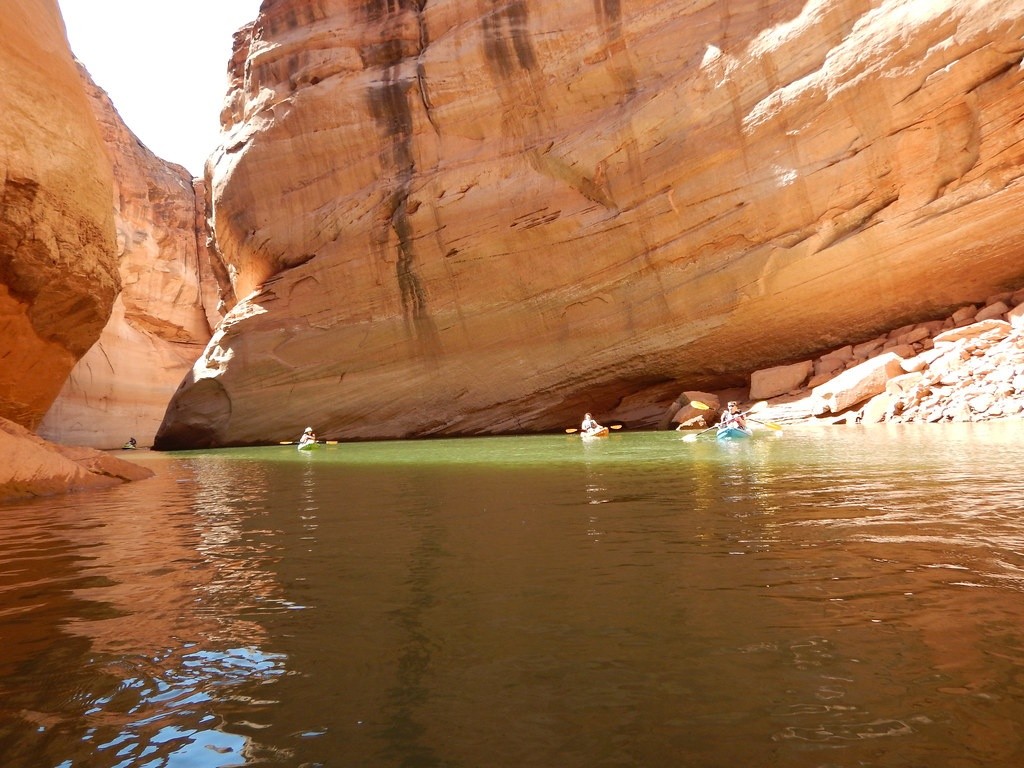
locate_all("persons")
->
[300,426,316,443]
[714,401,746,429]
[581,413,603,434]
[129,437,137,445]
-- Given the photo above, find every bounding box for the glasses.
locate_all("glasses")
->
[728,405,738,407]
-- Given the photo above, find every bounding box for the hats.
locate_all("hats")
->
[304,426,313,433]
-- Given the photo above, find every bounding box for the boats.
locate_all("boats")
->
[295,441,323,451]
[122,442,146,450]
[580,427,609,438]
[714,426,753,440]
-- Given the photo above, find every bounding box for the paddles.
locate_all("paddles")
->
[691,401,782,430]
[565,425,622,433]
[681,401,768,439]
[279,441,338,444]
